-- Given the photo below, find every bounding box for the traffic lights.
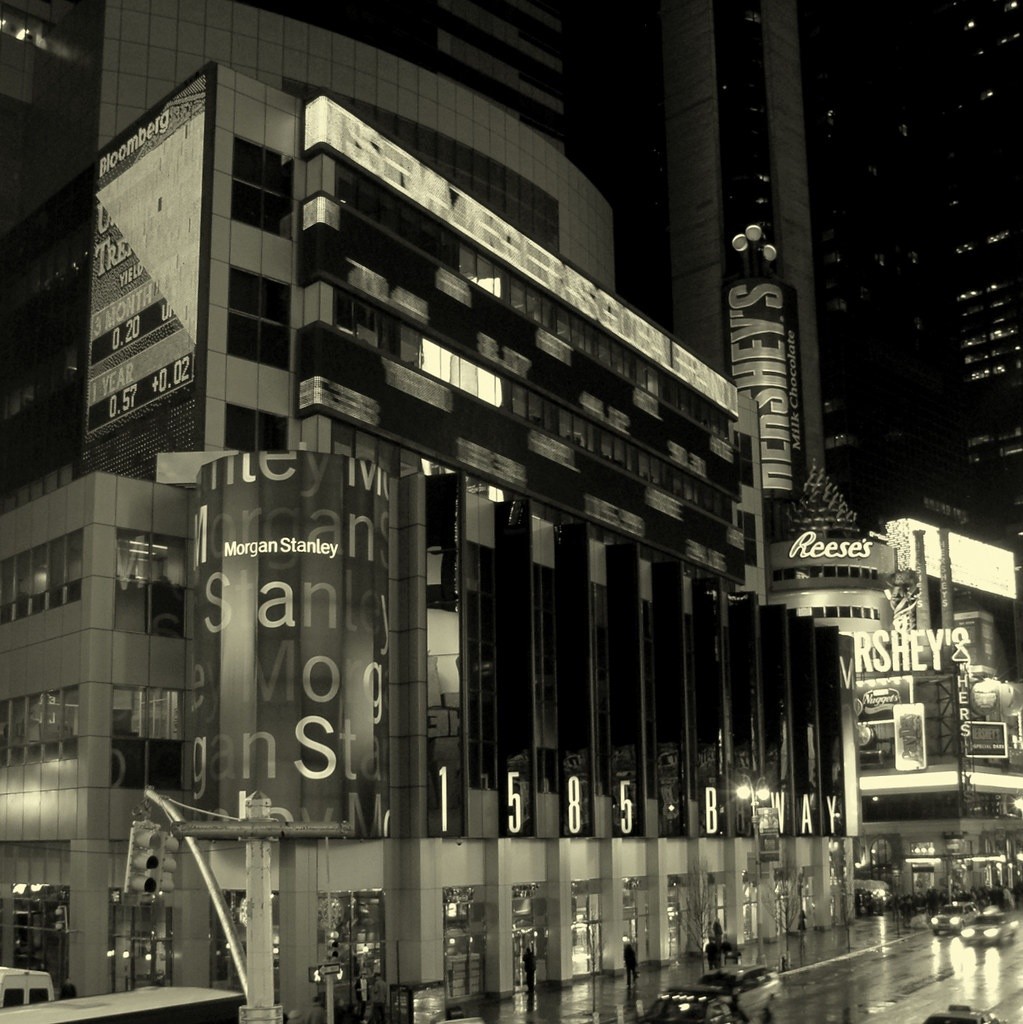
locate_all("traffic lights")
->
[123,820,179,894]
[328,939,338,958]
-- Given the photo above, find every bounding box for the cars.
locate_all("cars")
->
[931,904,980,935]
[960,916,1019,946]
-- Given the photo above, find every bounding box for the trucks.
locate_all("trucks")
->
[640,966,780,1024]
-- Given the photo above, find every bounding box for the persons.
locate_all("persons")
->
[370,972,388,1024]
[306,996,326,1024]
[354,972,368,1024]
[623,943,638,988]
[705,936,719,969]
[856,879,1023,928]
[706,918,723,952]
[522,947,537,994]
[798,909,808,931]
[60,975,76,999]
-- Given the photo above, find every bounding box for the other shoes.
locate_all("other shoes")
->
[633,974,637,980]
[626,982,629,985]
[525,990,533,993]
